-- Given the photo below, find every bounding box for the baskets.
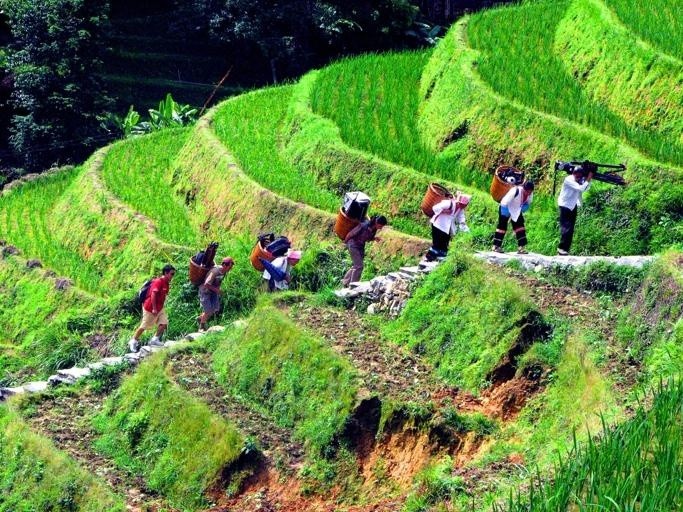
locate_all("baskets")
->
[190,255,216,286]
[489,165,524,203]
[333,207,371,240]
[250,239,276,272]
[421,183,453,218]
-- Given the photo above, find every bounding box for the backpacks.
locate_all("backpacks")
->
[138,281,151,303]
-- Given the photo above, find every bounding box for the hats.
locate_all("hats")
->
[223,257,234,263]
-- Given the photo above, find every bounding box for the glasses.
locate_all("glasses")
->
[170,272,174,276]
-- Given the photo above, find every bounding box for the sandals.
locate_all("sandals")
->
[493,246,504,253]
[518,249,528,255]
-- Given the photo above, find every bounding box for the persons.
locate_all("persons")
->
[423,192,472,262]
[263,249,302,292]
[493,181,534,254]
[558,166,593,255]
[196,257,235,334]
[128,265,177,354]
[341,215,387,290]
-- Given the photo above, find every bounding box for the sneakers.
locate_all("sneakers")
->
[128,340,137,352]
[150,336,164,346]
[198,328,206,333]
[557,247,568,256]
